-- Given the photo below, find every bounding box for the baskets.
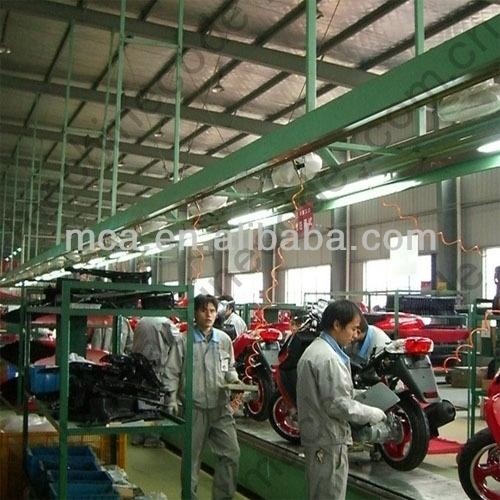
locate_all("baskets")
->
[7,363,77,394]
[26,446,120,500]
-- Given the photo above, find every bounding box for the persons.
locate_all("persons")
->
[296,300,391,500]
[91,290,247,500]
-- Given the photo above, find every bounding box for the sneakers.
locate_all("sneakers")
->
[131,440,164,448]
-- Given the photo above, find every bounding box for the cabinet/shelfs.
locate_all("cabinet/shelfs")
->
[235,303,311,324]
[304,291,476,455]
[20,280,194,500]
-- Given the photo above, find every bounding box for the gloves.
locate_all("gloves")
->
[164,402,178,416]
[370,407,386,425]
[233,380,245,386]
